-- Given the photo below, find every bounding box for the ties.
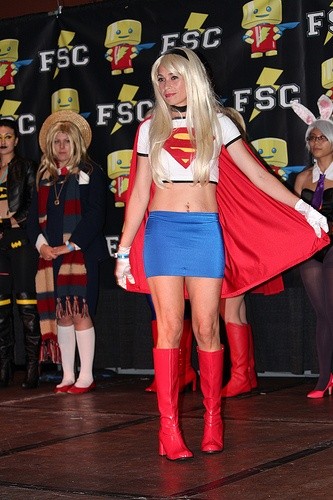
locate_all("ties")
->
[311,174,325,210]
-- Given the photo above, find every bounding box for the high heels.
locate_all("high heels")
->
[308,373,333,399]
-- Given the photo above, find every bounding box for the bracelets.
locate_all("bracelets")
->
[117,254,129,258]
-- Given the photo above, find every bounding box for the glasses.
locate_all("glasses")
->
[307,135,327,143]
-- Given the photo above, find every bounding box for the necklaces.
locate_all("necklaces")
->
[54,177,67,205]
[0,166,9,184]
[170,104,188,112]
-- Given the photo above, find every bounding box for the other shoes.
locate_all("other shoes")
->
[54,382,74,393]
[67,381,95,393]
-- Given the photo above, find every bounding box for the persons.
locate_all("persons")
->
[295,118,333,398]
[116,47,330,460]
[145,107,284,396]
[0,118,40,389]
[27,110,106,395]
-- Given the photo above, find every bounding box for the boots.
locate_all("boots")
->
[146,320,158,392]
[16,291,42,388]
[196,344,224,452]
[221,321,251,399]
[179,320,196,393]
[247,324,258,395]
[153,348,192,460]
[0,294,14,387]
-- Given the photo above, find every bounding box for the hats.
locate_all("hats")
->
[39,110,92,155]
